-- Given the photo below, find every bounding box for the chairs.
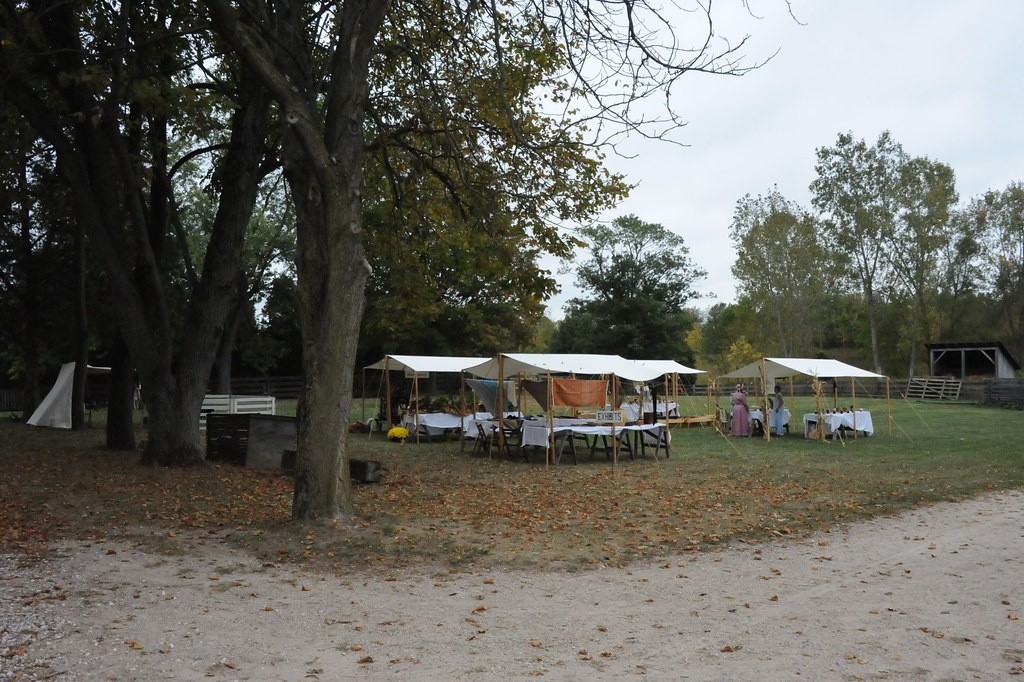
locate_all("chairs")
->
[500,428,527,463]
[476,422,499,456]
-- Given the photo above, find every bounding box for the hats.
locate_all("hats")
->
[734,384,743,390]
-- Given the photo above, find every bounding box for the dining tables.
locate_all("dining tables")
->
[463,418,596,449]
[520,423,672,466]
[801,410,873,440]
[606,402,680,418]
[401,412,525,443]
[729,409,792,437]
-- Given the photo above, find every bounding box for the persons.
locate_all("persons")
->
[771,385,784,436]
[731,384,750,437]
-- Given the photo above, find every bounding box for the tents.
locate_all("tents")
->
[716,357,891,440]
[362,355,492,445]
[460,353,664,466]
[613,359,710,424]
